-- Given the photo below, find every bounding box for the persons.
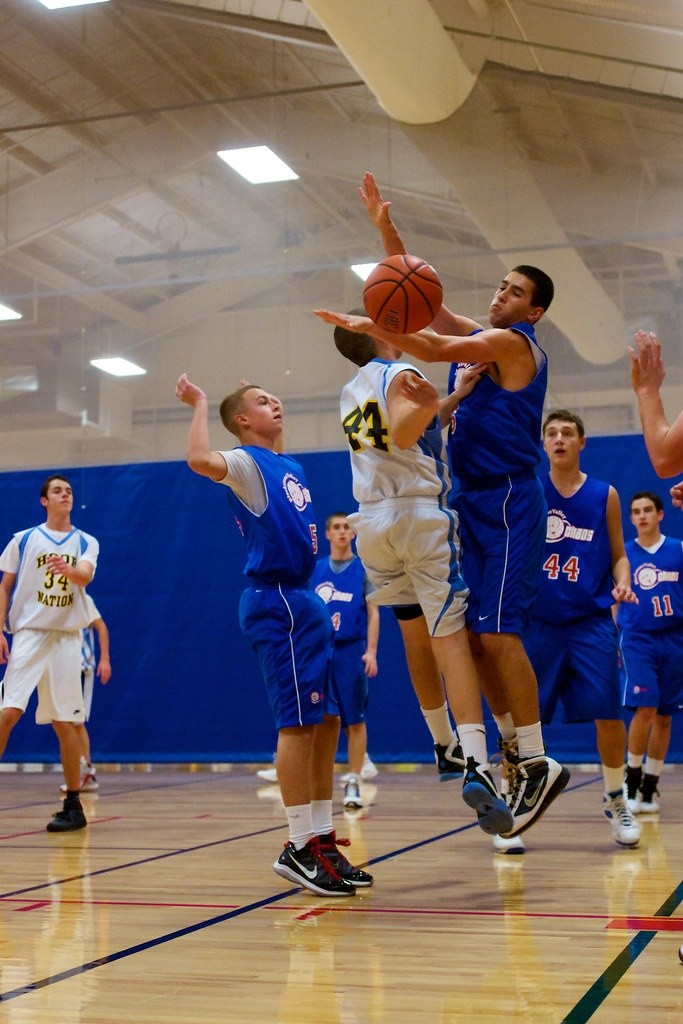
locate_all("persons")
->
[492,408,642,854]
[613,490,683,815]
[332,306,516,836]
[0,474,134,833]
[60,590,114,793]
[256,512,376,808]
[310,172,571,841]
[174,372,374,898]
[627,329,683,508]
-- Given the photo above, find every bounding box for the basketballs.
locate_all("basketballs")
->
[362,253,445,336]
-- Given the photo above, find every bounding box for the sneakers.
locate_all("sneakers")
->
[638,773,661,813]
[601,788,640,846]
[317,830,373,886]
[339,773,362,807]
[273,836,357,897]
[273,889,374,949]
[433,730,466,783]
[46,799,87,832]
[257,769,278,782]
[492,834,525,854]
[488,734,524,811]
[340,752,379,782]
[461,756,514,834]
[499,755,570,839]
[492,856,524,874]
[257,785,280,800]
[622,765,643,813]
[60,755,99,792]
